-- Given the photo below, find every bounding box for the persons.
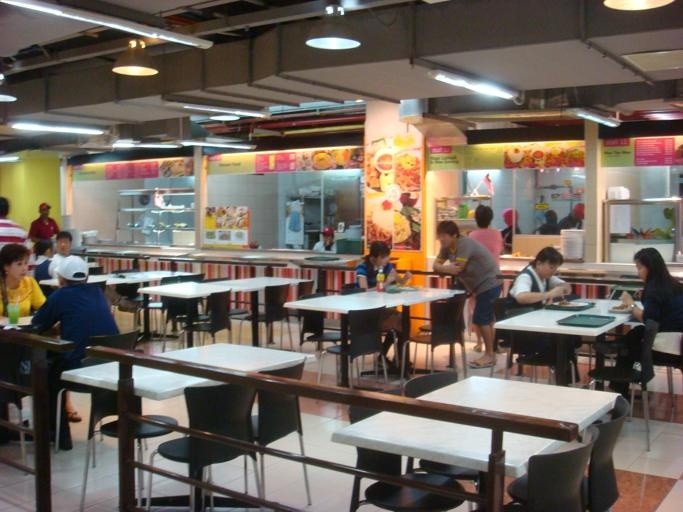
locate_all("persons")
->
[313,228,337,254]
[503,246,581,387]
[0,244,82,423]
[354,239,413,367]
[467,205,504,369]
[608,247,683,398]
[501,203,584,257]
[433,221,503,369]
[25,255,120,369]
[0,197,72,299]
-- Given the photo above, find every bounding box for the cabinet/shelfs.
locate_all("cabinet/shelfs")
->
[115,188,196,246]
[435,196,491,256]
[289,175,337,249]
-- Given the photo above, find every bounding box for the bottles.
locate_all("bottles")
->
[457,204,467,218]
[268,155,276,170]
[287,201,302,232]
[376,269,385,292]
[349,225,362,241]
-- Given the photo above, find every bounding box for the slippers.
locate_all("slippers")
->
[469,359,495,368]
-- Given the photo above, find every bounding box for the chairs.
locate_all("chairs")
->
[146,378,263,512]
[404,370,489,493]
[80,328,178,512]
[582,395,631,512]
[349,390,466,512]
[502,425,600,512]
[245,356,311,506]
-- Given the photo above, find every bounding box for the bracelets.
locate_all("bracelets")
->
[628,303,638,312]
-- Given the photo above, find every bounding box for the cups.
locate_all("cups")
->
[6,299,19,324]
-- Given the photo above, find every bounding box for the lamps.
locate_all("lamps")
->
[0,73,19,103]
[305,3,362,51]
[603,0,673,11]
[112,39,158,77]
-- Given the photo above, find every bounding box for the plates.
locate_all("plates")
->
[374,151,393,174]
[608,303,644,313]
[560,229,584,260]
[531,149,544,160]
[553,302,588,307]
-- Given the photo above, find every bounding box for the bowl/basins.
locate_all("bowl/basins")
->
[312,150,334,170]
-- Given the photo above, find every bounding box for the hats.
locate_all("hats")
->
[320,227,333,235]
[40,203,51,210]
[574,203,584,220]
[503,208,517,226]
[53,254,89,281]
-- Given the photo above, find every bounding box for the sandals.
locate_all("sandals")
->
[68,410,82,422]
